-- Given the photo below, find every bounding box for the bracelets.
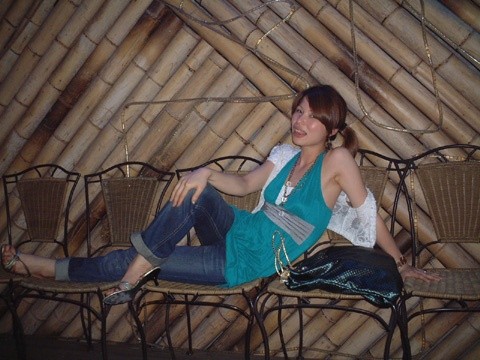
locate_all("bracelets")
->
[394,255,409,270]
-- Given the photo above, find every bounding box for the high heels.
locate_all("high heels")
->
[102,266,161,305]
[1,243,51,281]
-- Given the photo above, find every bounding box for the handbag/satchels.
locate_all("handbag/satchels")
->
[272,230,404,309]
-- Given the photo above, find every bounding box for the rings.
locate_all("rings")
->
[423,269,427,273]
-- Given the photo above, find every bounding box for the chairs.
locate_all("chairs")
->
[0,144,480,360]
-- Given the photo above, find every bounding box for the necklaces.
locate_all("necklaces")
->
[278,154,320,216]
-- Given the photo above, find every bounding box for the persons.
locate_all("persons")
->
[0,85,446,305]
